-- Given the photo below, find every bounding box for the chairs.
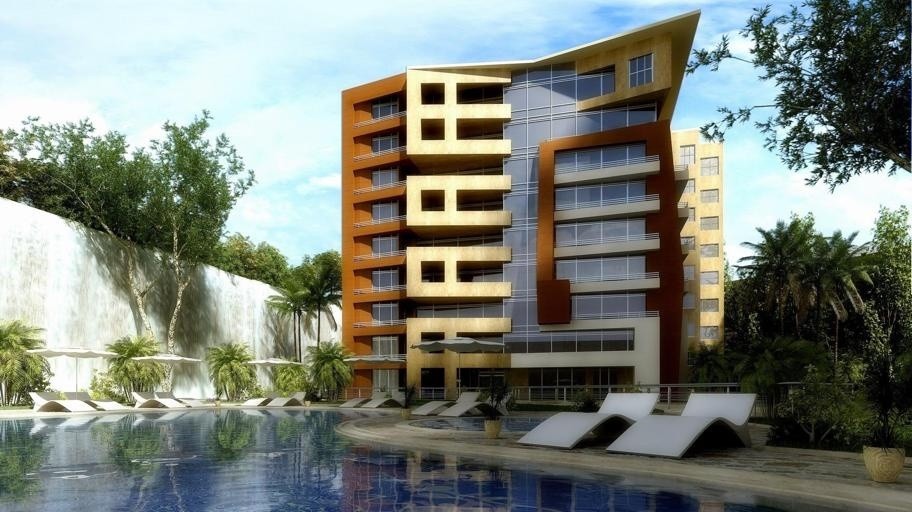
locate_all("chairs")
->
[411,392,512,417]
[241,392,306,407]
[339,391,406,408]
[28,391,192,412]
[516,393,759,458]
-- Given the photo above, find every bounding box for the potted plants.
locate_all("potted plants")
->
[485,373,512,439]
[401,384,415,420]
[857,345,911,483]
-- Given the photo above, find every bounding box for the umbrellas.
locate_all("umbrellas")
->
[20,430,118,485]
[26,346,120,400]
[342,357,404,391]
[242,357,305,391]
[224,418,296,464]
[127,423,202,470]
[131,352,203,391]
[407,337,507,398]
